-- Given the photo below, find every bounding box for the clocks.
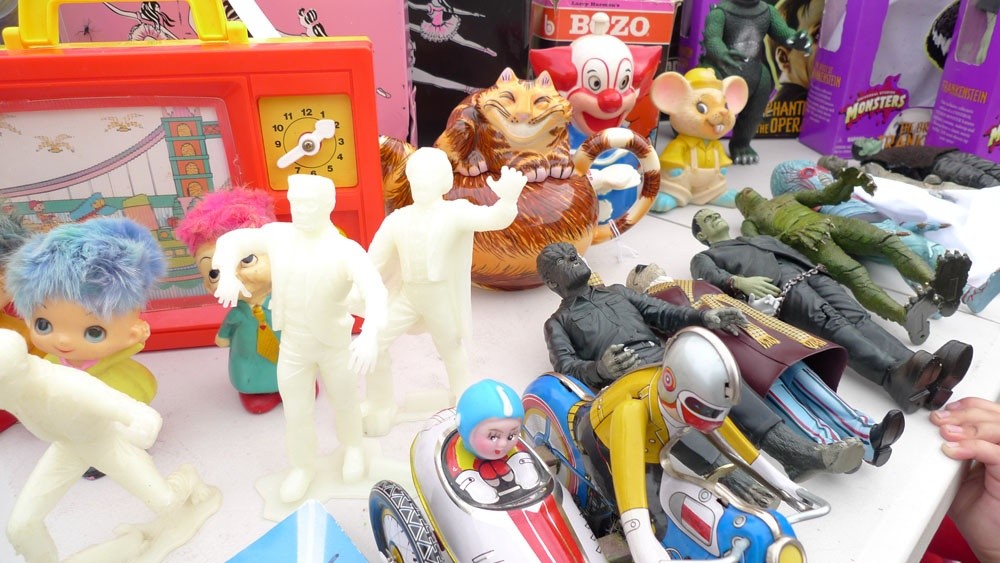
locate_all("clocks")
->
[257,88,363,192]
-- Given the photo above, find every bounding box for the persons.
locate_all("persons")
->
[443,379,538,506]
[919,395,999,562]
[735,166,971,344]
[179,185,281,415]
[211,170,391,501]
[355,145,528,437]
[853,137,1000,188]
[817,152,1000,255]
[691,208,974,416]
[627,257,905,473]
[578,325,804,562]
[538,241,862,516]
[8,220,156,479]
[0,328,218,563]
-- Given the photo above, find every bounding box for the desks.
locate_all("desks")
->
[0,121,999,562]
